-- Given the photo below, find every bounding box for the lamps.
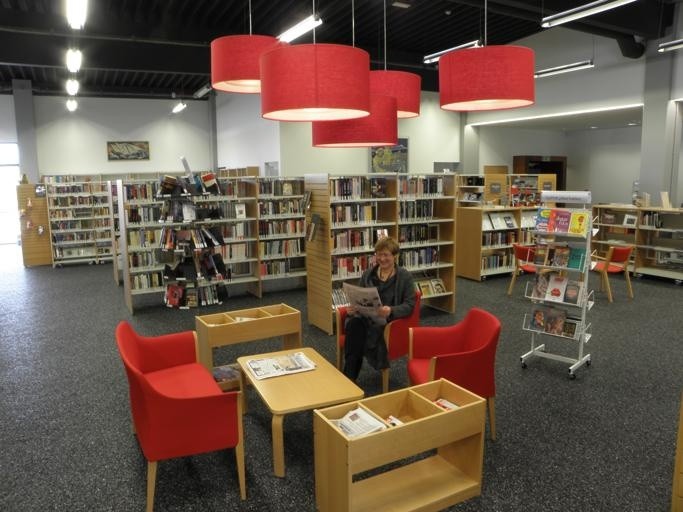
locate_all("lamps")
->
[658,39,683,52]
[312,0,397,148]
[439,0,535,112]
[259,0,370,121]
[423,40,485,63]
[276,12,323,42]
[542,1,637,27]
[534,60,594,79]
[193,83,213,99]
[369,0,421,118]
[212,0,291,94]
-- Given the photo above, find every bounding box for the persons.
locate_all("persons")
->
[342,237,417,382]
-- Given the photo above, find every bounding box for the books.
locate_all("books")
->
[47,175,119,259]
[385,415,404,429]
[331,286,351,311]
[400,246,441,270]
[330,201,380,228]
[532,268,580,305]
[259,238,301,258]
[533,242,587,271]
[535,206,590,236]
[642,211,664,228]
[123,172,255,310]
[327,407,387,440]
[301,190,312,210]
[399,223,441,247]
[258,199,303,216]
[482,231,516,250]
[259,218,305,238]
[257,178,305,197]
[261,257,306,276]
[399,199,434,222]
[331,253,377,280]
[399,174,444,197]
[409,270,448,298]
[305,223,316,242]
[331,228,389,255]
[527,302,582,337]
[432,397,462,413]
[481,251,516,273]
[329,175,390,202]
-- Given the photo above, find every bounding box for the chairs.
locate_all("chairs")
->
[115,320,245,512]
[406,309,501,441]
[336,289,421,392]
[591,246,636,303]
[507,244,537,296]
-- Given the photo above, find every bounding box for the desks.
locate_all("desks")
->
[236,347,364,478]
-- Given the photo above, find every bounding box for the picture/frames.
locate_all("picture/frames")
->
[434,161,460,173]
[367,137,409,173]
[107,141,150,161]
[265,161,279,176]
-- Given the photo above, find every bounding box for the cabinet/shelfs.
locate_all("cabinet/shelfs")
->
[589,203,682,282]
[44,166,260,182]
[116,177,308,315]
[519,190,600,381]
[313,378,484,512]
[196,303,303,366]
[108,181,123,286]
[457,206,538,282]
[455,174,557,208]
[304,174,456,335]
[16,181,113,268]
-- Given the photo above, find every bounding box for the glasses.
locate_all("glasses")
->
[374,252,391,258]
